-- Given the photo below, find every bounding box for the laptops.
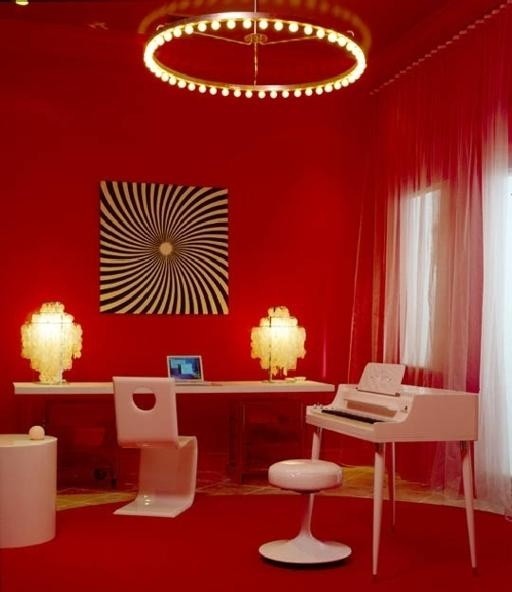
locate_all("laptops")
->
[167,355,223,387]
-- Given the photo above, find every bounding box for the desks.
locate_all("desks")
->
[9,379,337,404]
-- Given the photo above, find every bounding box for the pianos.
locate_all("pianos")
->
[306,362,479,574]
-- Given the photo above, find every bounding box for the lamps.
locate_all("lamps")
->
[250,305,307,383]
[20,301,85,385]
[142,0,367,101]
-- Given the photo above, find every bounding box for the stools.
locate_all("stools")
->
[258,458,352,566]
[0,434,60,551]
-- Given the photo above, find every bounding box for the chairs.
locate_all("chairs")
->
[109,376,199,519]
